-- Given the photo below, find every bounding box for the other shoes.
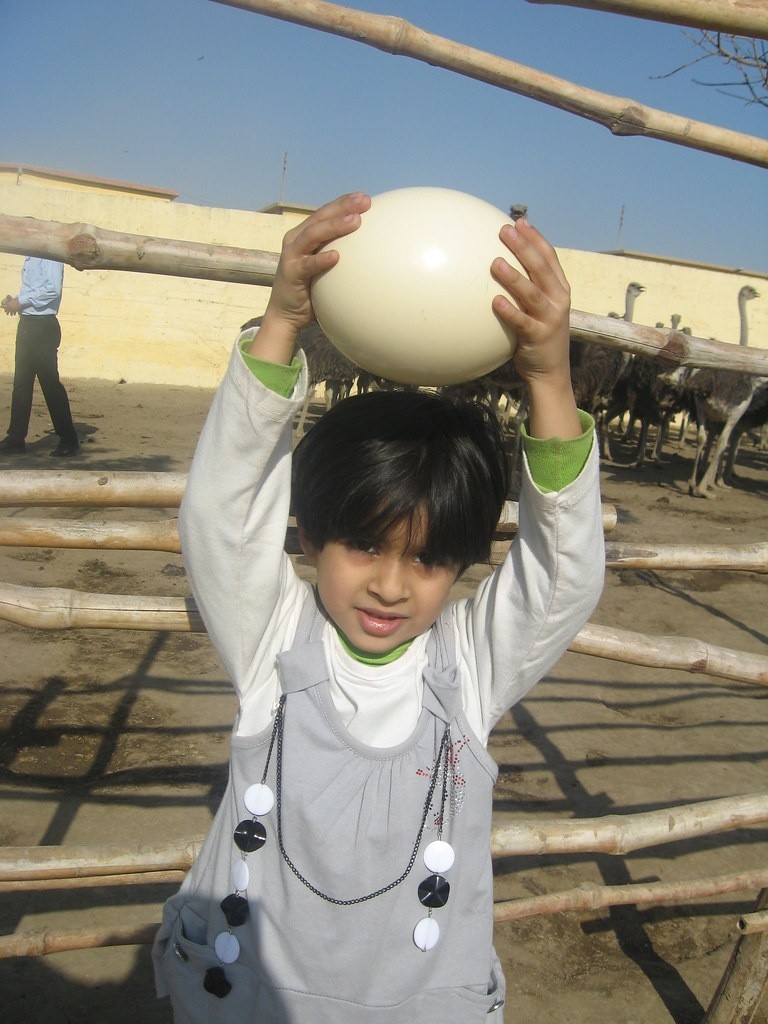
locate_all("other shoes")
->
[50,438,79,457]
[0,437,25,454]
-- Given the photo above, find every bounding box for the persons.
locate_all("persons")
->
[1,216,80,458]
[145,193,605,1024]
